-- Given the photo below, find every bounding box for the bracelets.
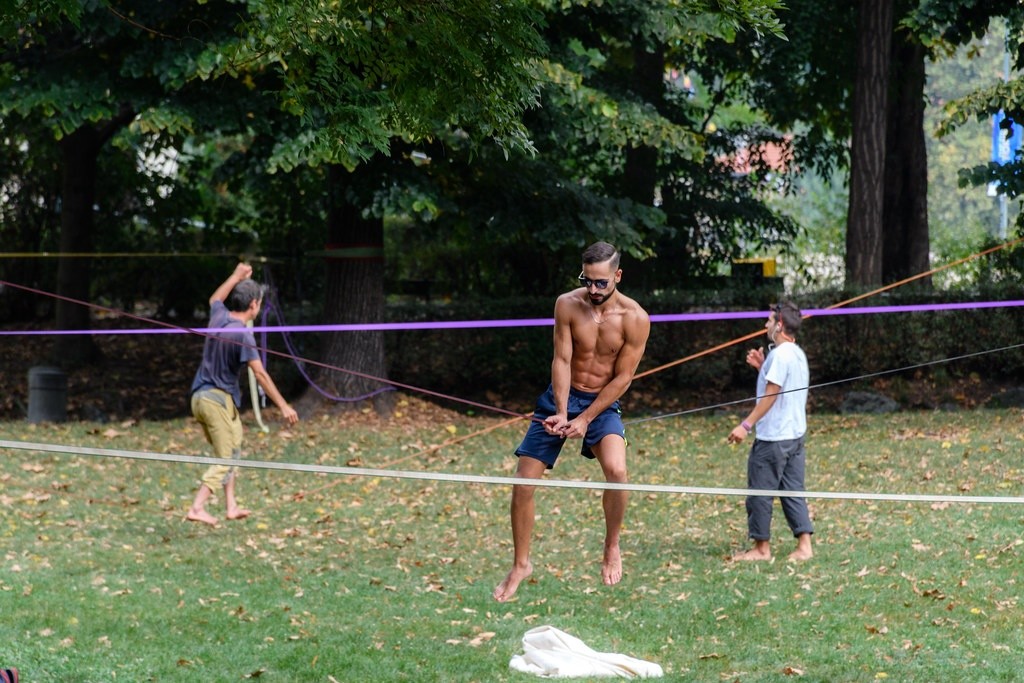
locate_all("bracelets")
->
[742,422,751,431]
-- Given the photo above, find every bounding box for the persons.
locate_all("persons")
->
[492,241,650,603]
[186,263,298,528]
[727,303,815,562]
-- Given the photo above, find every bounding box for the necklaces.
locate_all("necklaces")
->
[589,305,607,324]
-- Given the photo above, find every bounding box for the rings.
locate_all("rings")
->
[733,440,736,444]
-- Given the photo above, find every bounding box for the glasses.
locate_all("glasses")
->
[578,270,615,289]
[776,303,783,325]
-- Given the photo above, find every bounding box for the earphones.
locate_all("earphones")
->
[775,325,781,332]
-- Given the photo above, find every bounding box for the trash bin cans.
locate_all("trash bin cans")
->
[29,368,68,425]
